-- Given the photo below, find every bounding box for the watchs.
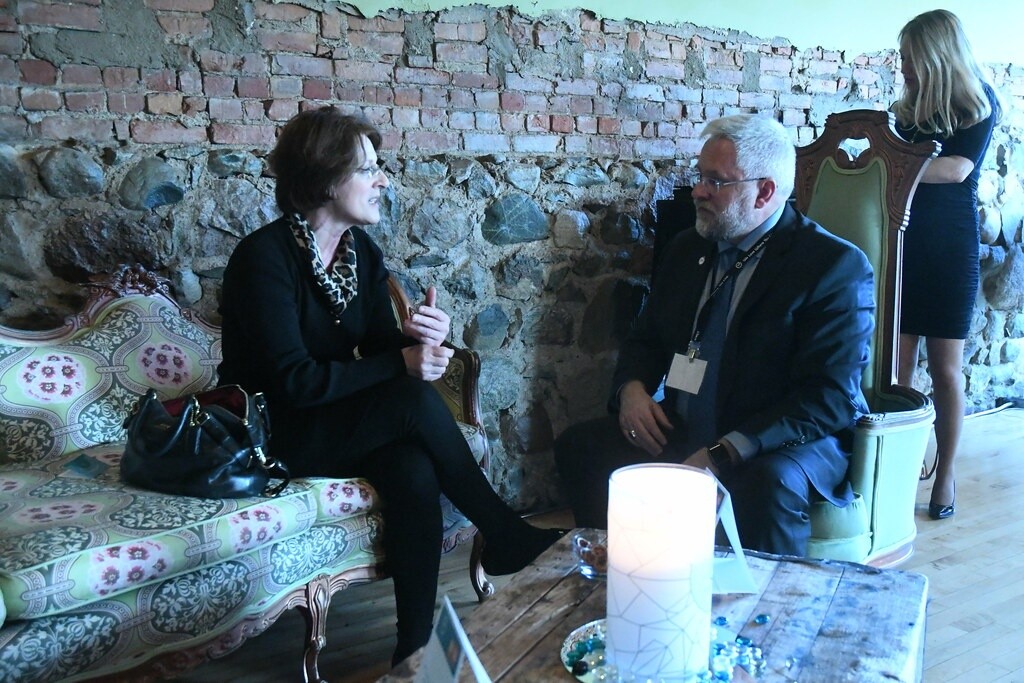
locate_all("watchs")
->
[704,439,734,478]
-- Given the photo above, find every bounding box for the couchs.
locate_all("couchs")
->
[0,260,497,683]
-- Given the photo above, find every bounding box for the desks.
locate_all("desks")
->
[381,528,930,683]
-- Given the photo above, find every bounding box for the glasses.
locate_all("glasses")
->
[343,161,384,179]
[693,171,767,195]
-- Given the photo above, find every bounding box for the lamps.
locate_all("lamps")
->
[606,462,717,683]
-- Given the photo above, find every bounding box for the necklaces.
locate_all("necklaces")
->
[899,123,921,142]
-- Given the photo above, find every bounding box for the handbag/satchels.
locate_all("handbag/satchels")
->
[120,384,291,500]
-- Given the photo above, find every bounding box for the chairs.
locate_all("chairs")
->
[786,110,943,571]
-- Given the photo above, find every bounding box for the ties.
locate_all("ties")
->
[673,248,748,463]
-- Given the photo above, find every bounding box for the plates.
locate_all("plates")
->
[559,619,606,683]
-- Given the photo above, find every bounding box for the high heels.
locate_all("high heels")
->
[929,478,956,520]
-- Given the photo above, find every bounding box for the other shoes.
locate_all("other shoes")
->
[479,527,574,575]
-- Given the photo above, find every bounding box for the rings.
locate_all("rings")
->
[628,429,637,440]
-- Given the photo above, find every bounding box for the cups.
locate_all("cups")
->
[574,530,607,582]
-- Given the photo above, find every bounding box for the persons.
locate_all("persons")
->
[214,107,576,677]
[569,113,878,560]
[886,5,998,521]
